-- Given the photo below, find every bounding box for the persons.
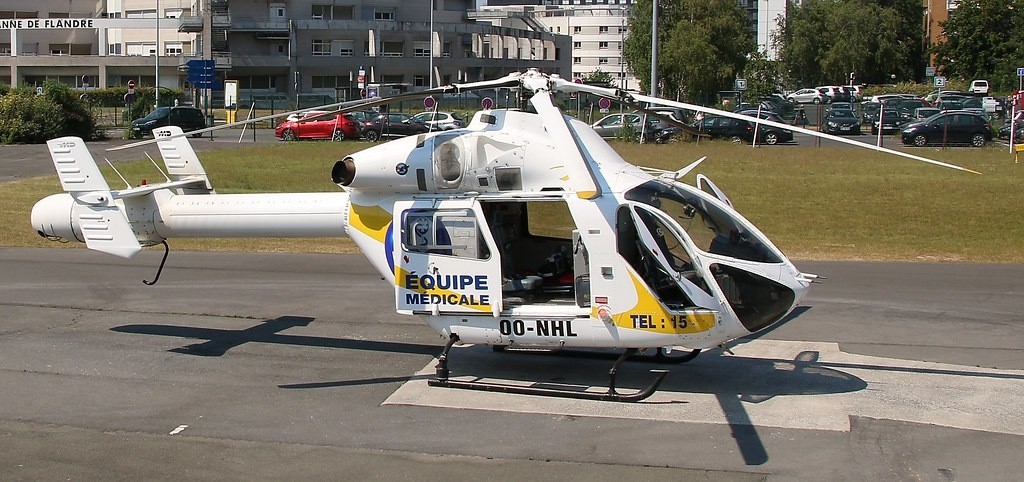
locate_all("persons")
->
[1000,94,1012,118]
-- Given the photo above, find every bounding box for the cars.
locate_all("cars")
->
[822,102,861,135]
[736,110,793,145]
[690,116,758,145]
[815,84,865,103]
[732,93,800,126]
[861,80,1024,143]
[786,89,827,105]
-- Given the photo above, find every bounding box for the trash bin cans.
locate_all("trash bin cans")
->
[207,114,214,125]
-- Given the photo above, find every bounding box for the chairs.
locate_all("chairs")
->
[490,209,542,291]
[635,231,700,296]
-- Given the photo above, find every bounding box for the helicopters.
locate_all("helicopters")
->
[31,67,983,402]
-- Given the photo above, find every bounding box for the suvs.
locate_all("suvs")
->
[402,112,465,132]
[362,112,429,143]
[590,113,667,144]
[275,111,361,142]
[901,110,995,147]
[131,106,206,139]
[611,107,688,139]
